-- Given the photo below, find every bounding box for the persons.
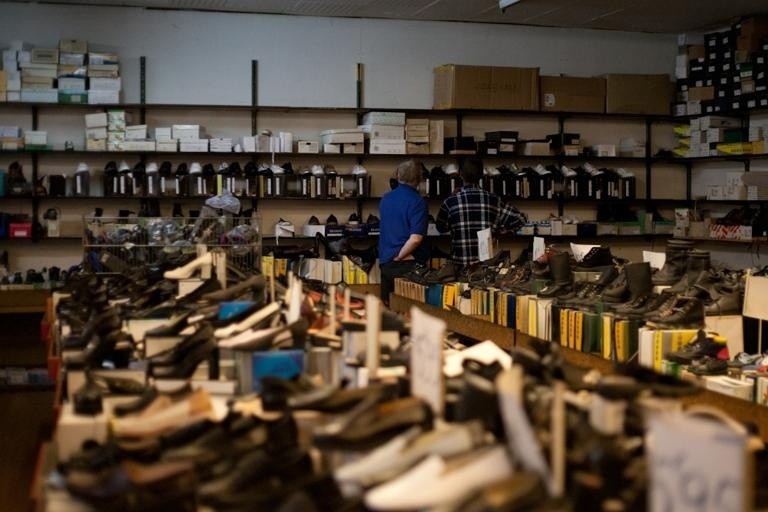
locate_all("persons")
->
[434,154,528,268]
[378,157,429,303]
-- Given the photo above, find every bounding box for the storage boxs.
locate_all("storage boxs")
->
[670,16,768,120]
[0,37,121,104]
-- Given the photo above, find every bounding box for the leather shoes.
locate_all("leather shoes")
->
[716,205,768,225]
[105,160,366,176]
[3,267,59,283]
[421,162,633,178]
[84,198,259,255]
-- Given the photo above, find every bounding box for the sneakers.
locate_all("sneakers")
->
[308,216,319,225]
[368,215,379,224]
[572,216,580,224]
[44,207,56,218]
[326,215,336,226]
[561,215,571,224]
[349,214,359,224]
[279,218,290,225]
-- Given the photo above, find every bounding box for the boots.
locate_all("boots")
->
[8,162,26,195]
[32,177,46,197]
